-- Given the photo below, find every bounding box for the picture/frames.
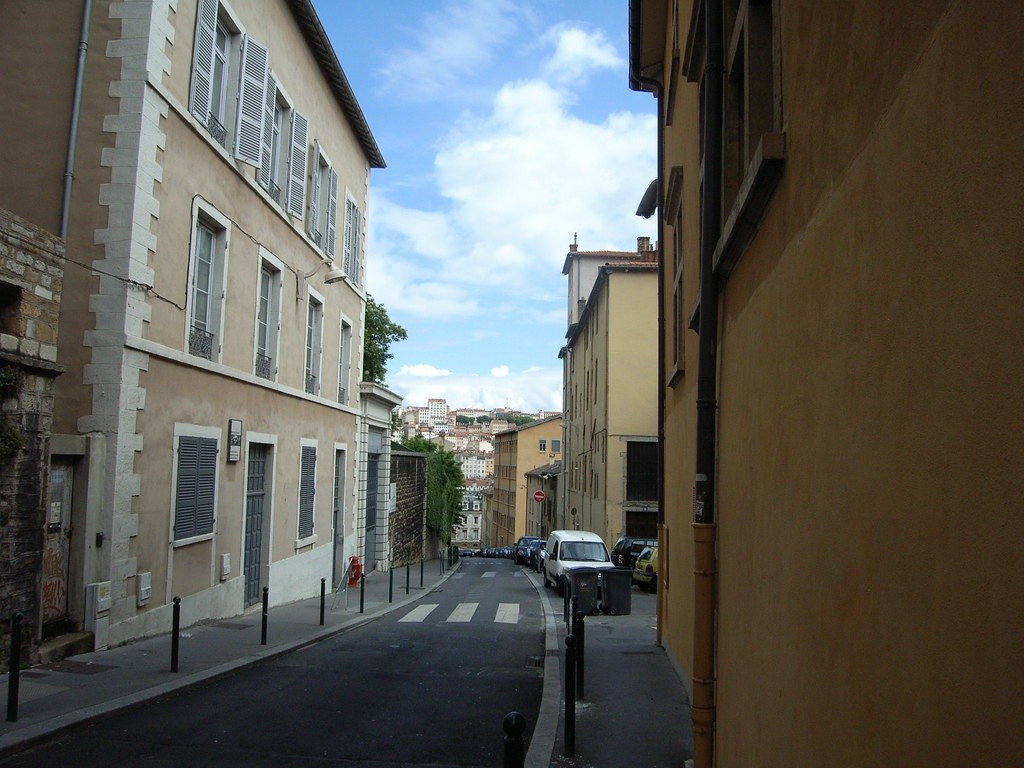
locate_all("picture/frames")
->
[227,419,242,462]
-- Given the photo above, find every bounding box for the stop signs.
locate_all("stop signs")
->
[534,491,545,502]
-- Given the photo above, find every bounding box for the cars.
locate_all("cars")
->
[525,540,548,571]
[632,545,659,595]
[458,546,513,559]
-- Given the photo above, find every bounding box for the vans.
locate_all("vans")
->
[543,529,615,600]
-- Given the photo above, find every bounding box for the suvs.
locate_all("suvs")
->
[513,536,544,565]
[611,535,659,570]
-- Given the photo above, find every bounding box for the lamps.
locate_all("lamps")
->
[306,260,348,284]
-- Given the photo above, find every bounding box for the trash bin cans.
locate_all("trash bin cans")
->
[597,567,634,616]
[567,567,600,616]
